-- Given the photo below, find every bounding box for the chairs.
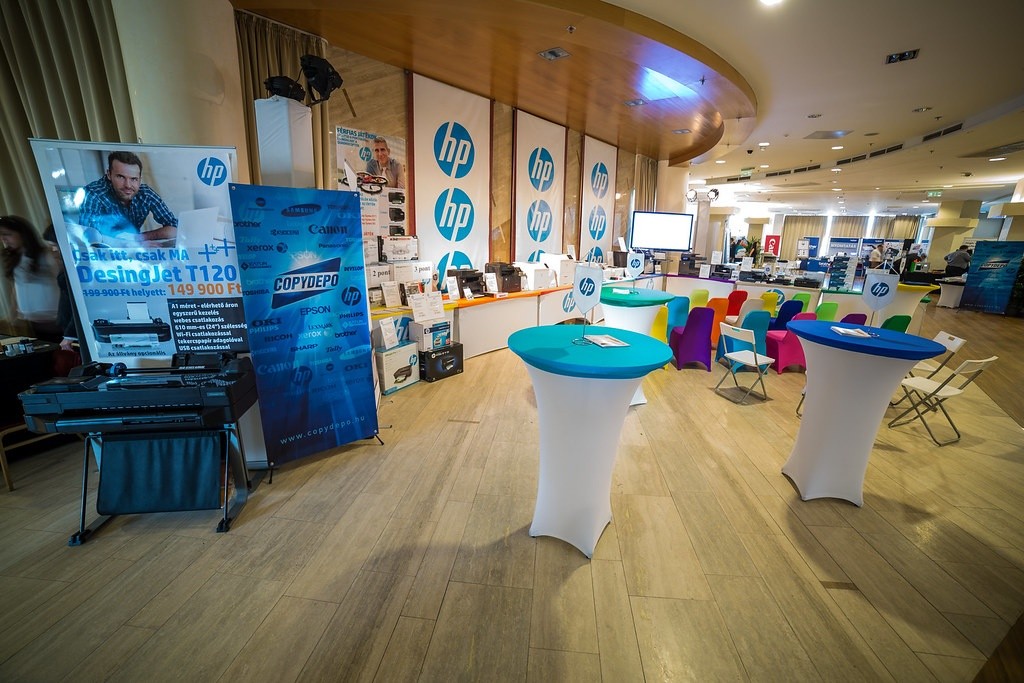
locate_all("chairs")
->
[650,289,999,446]
[856,259,864,276]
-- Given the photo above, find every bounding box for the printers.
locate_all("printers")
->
[389,192,405,236]
[486,261,522,292]
[17,350,261,437]
[739,270,769,284]
[92,309,172,347]
[393,364,413,384]
[448,268,486,297]
[441,356,454,370]
[709,264,735,279]
[677,252,707,276]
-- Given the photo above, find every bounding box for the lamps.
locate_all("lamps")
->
[264,54,344,106]
[686,189,720,203]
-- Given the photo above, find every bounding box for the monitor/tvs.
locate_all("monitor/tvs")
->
[631,211,693,251]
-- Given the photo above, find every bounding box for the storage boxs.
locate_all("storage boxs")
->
[371,318,464,413]
[367,236,434,304]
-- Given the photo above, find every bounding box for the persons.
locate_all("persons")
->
[943,245,972,275]
[0,215,61,339]
[366,136,406,188]
[78,150,179,249]
[749,242,758,264]
[43,221,79,376]
[869,244,883,269]
[889,253,927,275]
[729,238,747,263]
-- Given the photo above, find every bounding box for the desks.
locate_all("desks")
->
[903,271,946,294]
[665,273,941,337]
[0,333,86,492]
[782,320,947,507]
[508,324,674,560]
[601,286,675,407]
[369,270,664,361]
[935,279,966,309]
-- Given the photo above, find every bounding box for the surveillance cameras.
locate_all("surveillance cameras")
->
[747,150,753,155]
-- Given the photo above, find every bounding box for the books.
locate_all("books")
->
[584,334,629,347]
[830,326,871,337]
